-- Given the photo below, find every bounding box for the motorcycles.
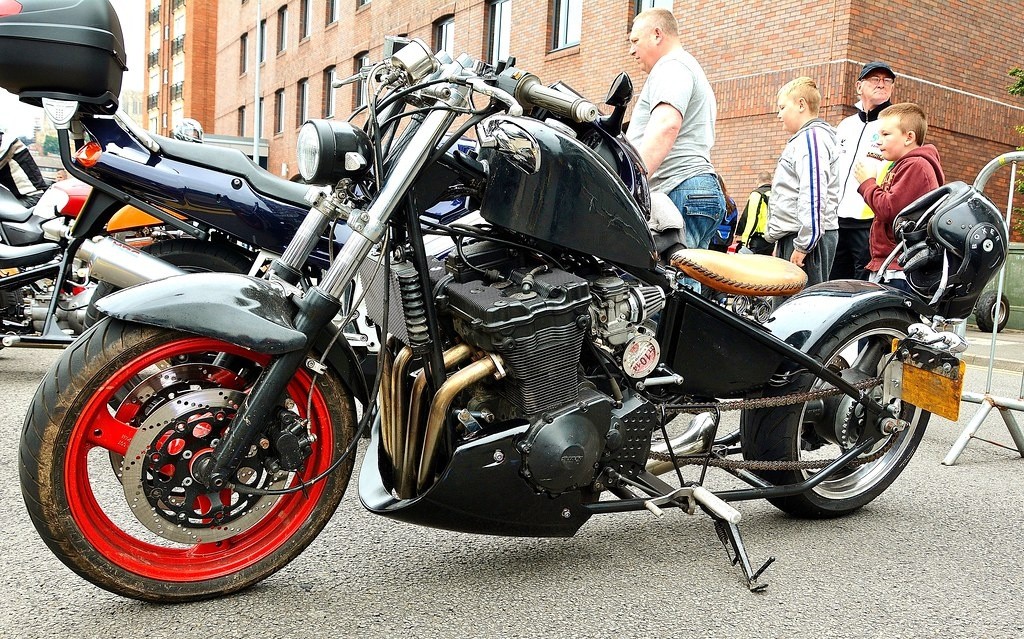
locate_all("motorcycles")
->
[0,0,693,351]
[16,36,1010,603]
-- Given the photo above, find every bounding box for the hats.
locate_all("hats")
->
[857,62,896,84]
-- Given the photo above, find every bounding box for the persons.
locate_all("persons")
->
[765,77,839,311]
[172,118,203,143]
[627,8,725,295]
[701,176,737,302]
[829,63,896,355]
[736,171,774,257]
[855,103,945,294]
[57,170,67,182]
[0,130,49,208]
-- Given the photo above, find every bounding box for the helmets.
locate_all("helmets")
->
[892,180,1008,302]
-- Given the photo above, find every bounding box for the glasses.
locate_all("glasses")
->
[861,76,894,86]
